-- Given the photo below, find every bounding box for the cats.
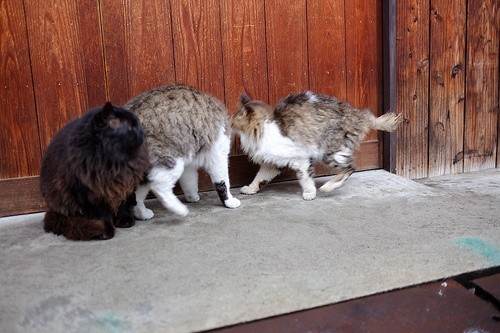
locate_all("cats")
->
[40,99,154,241]
[120,83,242,222]
[229,89,404,201]
